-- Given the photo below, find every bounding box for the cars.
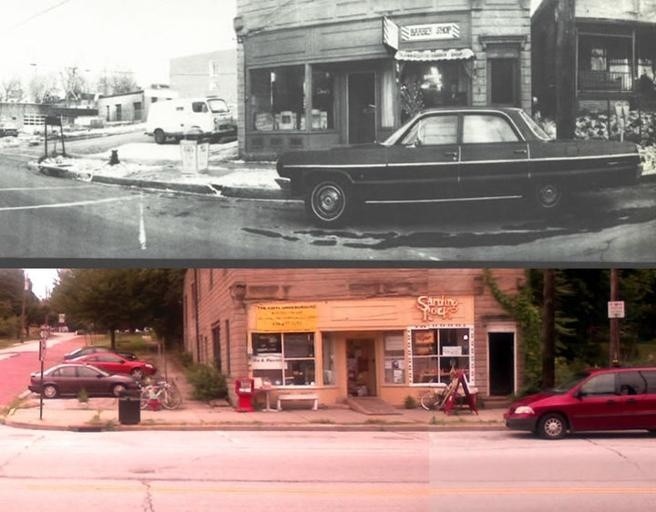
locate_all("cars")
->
[0,122,18,137]
[503,366,656,440]
[274,105,645,229]
[27,343,157,399]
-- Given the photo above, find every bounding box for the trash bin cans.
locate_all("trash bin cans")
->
[119,390,141,424]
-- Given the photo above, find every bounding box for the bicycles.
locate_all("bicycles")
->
[420,380,469,410]
[135,375,182,410]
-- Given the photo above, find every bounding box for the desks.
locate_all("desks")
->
[254,385,278,412]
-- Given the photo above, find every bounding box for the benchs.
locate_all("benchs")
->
[277,394,319,411]
[434,386,479,409]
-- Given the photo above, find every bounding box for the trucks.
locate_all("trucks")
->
[143,97,237,144]
